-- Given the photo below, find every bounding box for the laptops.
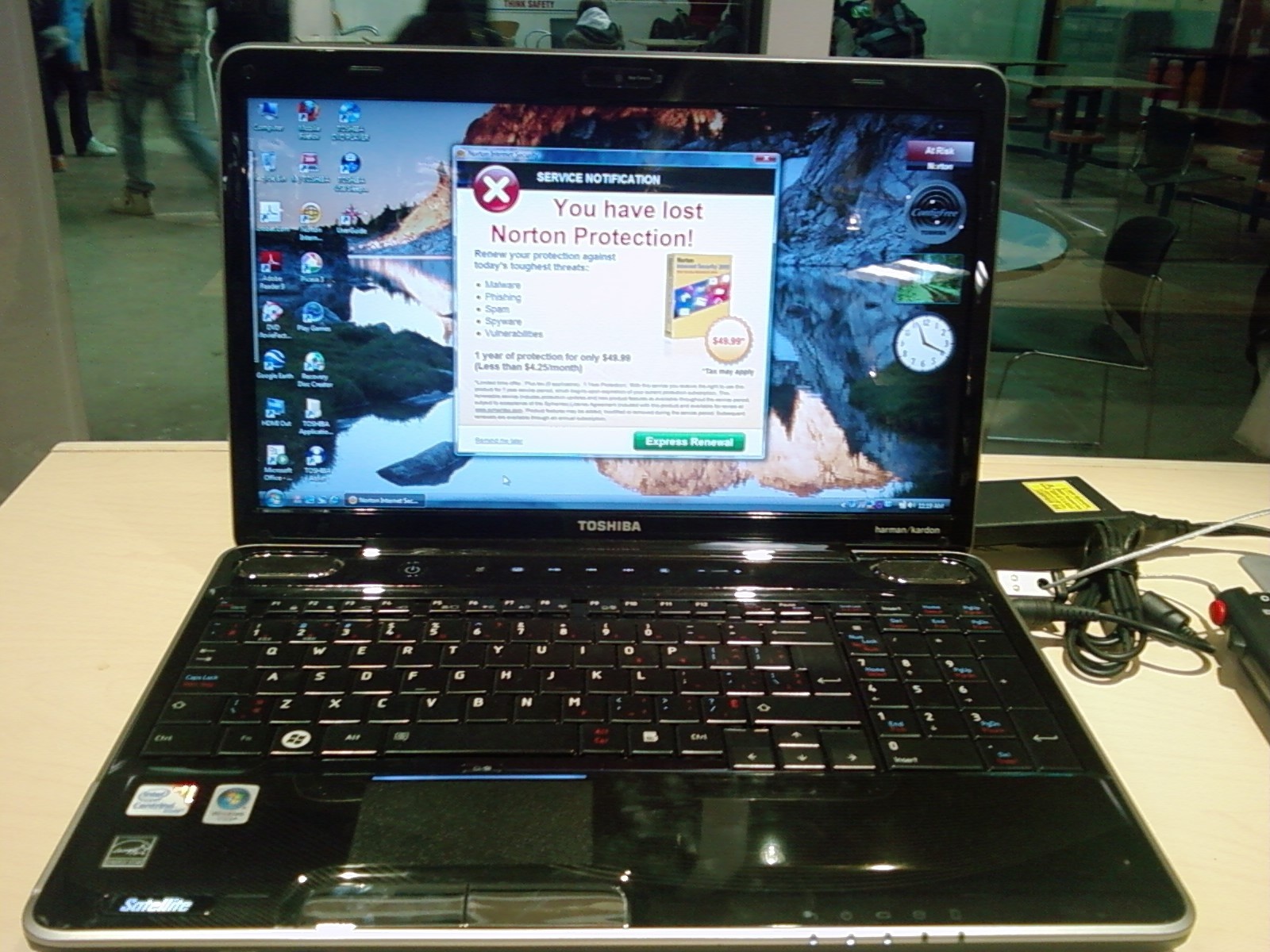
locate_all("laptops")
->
[18,41,1194,951]
[550,18,578,49]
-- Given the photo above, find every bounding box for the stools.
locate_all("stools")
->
[1006,97,1270,218]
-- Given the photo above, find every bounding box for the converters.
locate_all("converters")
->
[972,476,1130,552]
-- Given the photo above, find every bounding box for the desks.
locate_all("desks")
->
[1004,76,1175,170]
[627,37,707,49]
[991,211,1069,286]
[926,54,1069,133]
[0,440,1269,952]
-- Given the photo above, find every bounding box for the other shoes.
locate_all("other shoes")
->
[79,137,117,158]
[50,154,68,173]
[107,188,158,218]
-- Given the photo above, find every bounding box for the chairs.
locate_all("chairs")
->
[982,212,1180,460]
[331,10,551,49]
[1109,104,1246,239]
[489,21,520,49]
[525,29,551,49]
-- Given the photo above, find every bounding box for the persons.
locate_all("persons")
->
[397,0,502,47]
[834,0,927,58]
[102,0,290,215]
[28,0,117,170]
[688,0,763,53]
[563,0,625,50]
